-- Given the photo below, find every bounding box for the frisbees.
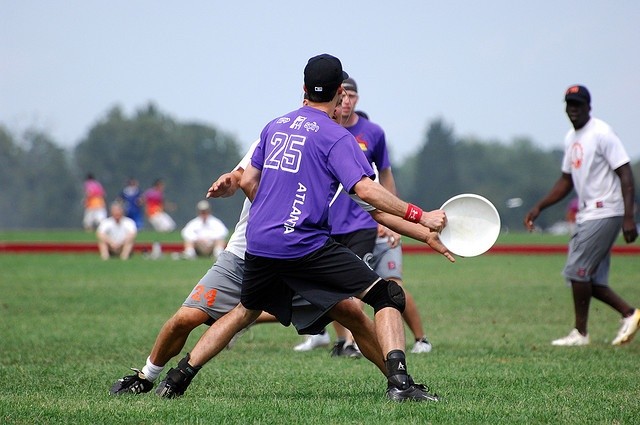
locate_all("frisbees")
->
[437,193,501,258]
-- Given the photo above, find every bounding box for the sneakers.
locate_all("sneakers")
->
[293,331,330,351]
[612,309,640,345]
[387,375,430,396]
[410,337,432,353]
[156,353,202,399]
[112,368,154,394]
[552,328,590,346]
[383,350,440,402]
[331,340,363,358]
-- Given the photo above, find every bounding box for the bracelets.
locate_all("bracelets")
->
[402,203,424,224]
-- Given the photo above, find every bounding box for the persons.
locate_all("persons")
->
[138,178,177,235]
[293,111,434,354]
[108,87,391,396]
[182,198,228,258]
[330,320,361,357]
[524,83,640,347]
[120,176,145,230]
[82,174,107,231]
[327,76,399,359]
[155,52,448,403]
[96,201,138,260]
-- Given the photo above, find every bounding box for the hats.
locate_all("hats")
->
[304,54,348,91]
[566,87,590,104]
[341,77,357,92]
